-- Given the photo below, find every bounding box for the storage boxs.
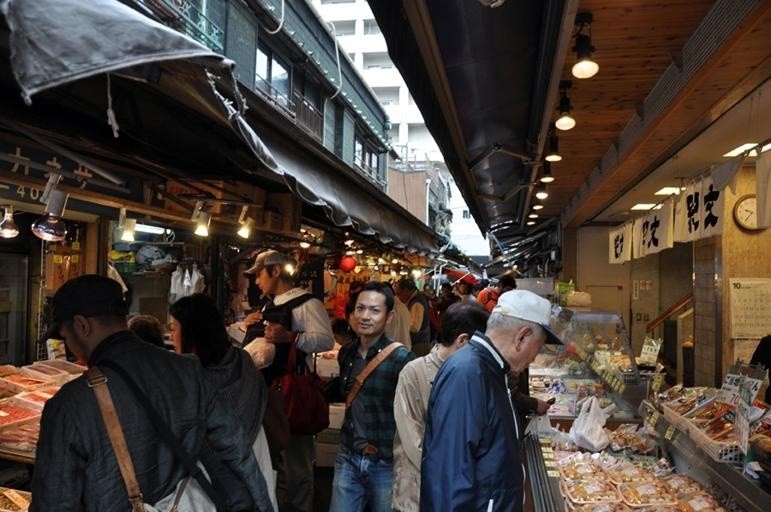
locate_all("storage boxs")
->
[15,390,52,411]
[35,358,88,374]
[514,277,553,297]
[1,396,41,432]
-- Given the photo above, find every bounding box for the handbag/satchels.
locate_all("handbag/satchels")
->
[91,360,259,512]
[270,329,329,436]
[86,366,217,512]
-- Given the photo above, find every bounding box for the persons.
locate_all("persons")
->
[394,279,431,357]
[509,373,551,425]
[332,319,355,346]
[28,274,275,512]
[348,281,364,293]
[242,250,334,512]
[748,335,771,406]
[479,279,490,293]
[384,281,413,351]
[391,304,490,512]
[127,314,164,347]
[477,274,517,314]
[460,282,476,305]
[419,290,564,512]
[169,294,278,511]
[330,281,417,512]
[423,282,433,292]
[425,289,440,318]
[408,279,438,340]
[344,292,360,337]
[437,283,461,316]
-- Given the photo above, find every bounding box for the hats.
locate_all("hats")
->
[36,274,128,344]
[243,251,288,278]
[492,289,564,346]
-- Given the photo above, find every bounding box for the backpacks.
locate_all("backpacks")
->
[241,293,316,389]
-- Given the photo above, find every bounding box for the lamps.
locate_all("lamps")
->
[32,189,70,241]
[573,10,600,79]
[546,123,562,162]
[191,201,212,237]
[1,204,19,239]
[120,219,135,242]
[556,80,578,130]
[236,205,255,239]
[535,183,548,199]
[539,162,555,183]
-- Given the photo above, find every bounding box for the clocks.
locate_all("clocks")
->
[733,193,758,232]
